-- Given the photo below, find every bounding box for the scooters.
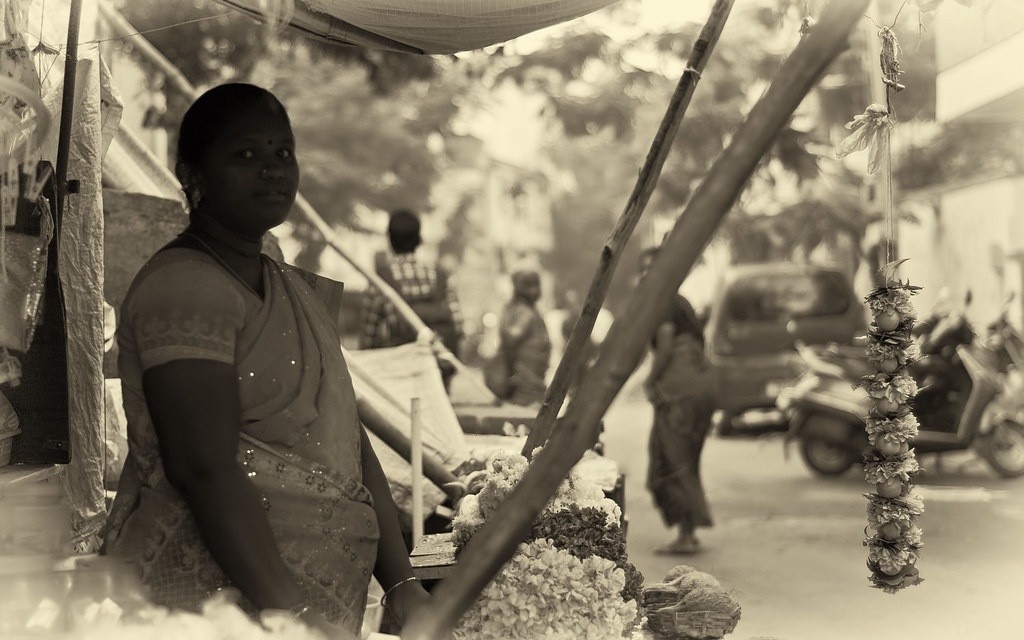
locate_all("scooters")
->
[771,288,1023,478]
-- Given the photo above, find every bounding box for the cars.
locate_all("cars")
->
[696,264,865,436]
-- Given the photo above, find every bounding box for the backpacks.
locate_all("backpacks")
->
[375,257,457,376]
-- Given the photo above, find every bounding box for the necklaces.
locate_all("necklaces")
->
[177,232,262,301]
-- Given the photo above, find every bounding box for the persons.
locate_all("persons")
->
[500,269,552,406]
[361,210,463,393]
[97,84,434,640]
[636,247,716,555]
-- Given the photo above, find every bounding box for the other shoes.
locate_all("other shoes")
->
[654,540,701,554]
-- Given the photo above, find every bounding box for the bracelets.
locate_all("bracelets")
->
[381,576,420,607]
[295,605,310,618]
